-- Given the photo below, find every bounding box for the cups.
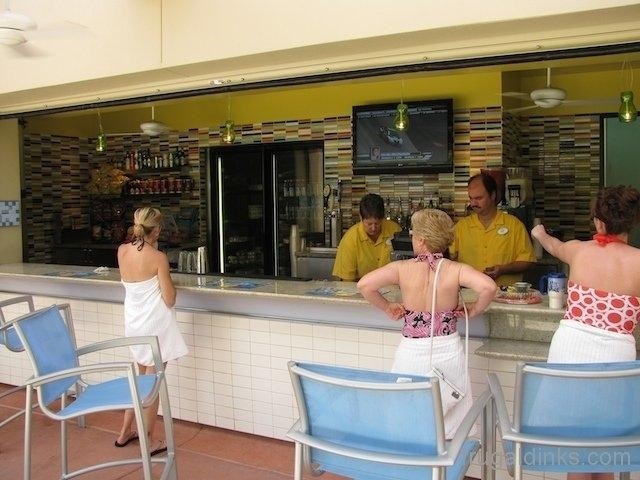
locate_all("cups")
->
[127,176,192,195]
[289,223,305,279]
[517,283,527,295]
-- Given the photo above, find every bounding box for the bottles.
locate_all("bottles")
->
[384,199,435,236]
[281,178,307,196]
[168,246,209,276]
[123,146,187,171]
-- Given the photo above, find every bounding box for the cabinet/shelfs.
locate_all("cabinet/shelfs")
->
[81,165,191,243]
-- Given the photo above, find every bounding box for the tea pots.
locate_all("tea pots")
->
[539,273,569,309]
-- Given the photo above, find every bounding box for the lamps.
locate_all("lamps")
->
[95,108,108,153]
[0,0,37,47]
[139,107,168,137]
[618,53,638,123]
[529,58,568,110]
[394,77,410,131]
[221,92,235,143]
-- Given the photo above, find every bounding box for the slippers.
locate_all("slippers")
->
[115,429,139,449]
[139,438,168,459]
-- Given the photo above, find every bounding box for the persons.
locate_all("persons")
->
[332,194,402,282]
[114,205,188,456]
[357,208,497,440]
[531,184,639,364]
[445,173,537,287]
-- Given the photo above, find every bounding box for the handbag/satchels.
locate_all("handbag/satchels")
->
[422,364,467,421]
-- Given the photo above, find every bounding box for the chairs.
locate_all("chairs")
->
[13,305,178,480]
[286,360,495,480]
[486,359,640,480]
[0,296,86,428]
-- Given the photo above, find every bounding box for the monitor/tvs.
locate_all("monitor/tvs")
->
[353,98,453,175]
[390,231,451,260]
